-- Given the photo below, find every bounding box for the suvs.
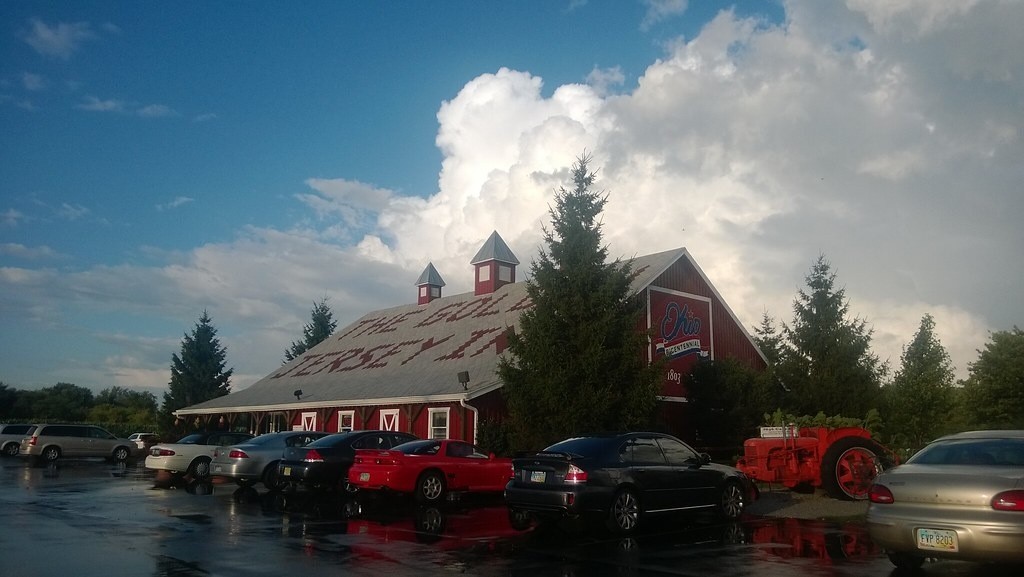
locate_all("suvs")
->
[127,431,162,448]
[0,424,38,457]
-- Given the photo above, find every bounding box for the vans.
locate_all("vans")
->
[19,422,137,462]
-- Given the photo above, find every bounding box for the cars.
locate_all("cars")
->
[208,428,330,490]
[865,431,1024,570]
[274,426,426,494]
[348,437,516,500]
[509,521,750,557]
[145,429,256,475]
[348,500,535,566]
[502,432,749,534]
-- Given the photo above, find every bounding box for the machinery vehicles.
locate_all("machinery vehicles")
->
[737,418,901,502]
